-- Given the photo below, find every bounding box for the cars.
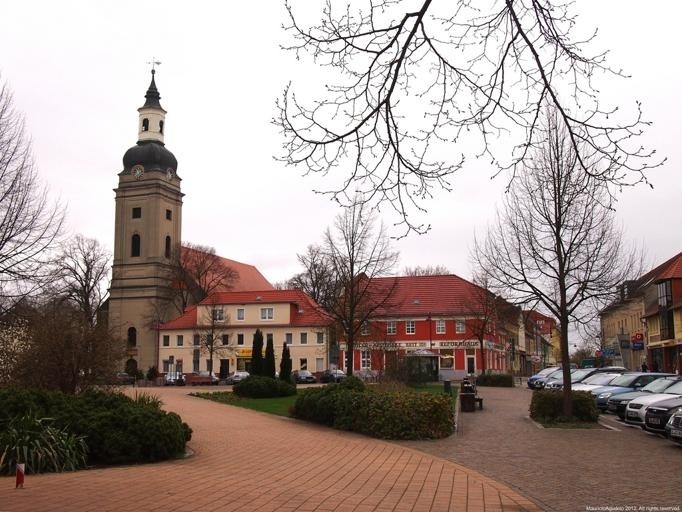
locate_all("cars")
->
[225,371,249,385]
[110,372,133,384]
[528,363,682,444]
[320,370,346,383]
[192,371,219,385]
[155,372,185,385]
[291,370,316,384]
[354,370,377,383]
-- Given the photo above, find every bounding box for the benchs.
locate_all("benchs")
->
[191,377,212,385]
[460,377,483,412]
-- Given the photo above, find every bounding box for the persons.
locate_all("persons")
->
[652,361,658,372]
[641,362,649,372]
